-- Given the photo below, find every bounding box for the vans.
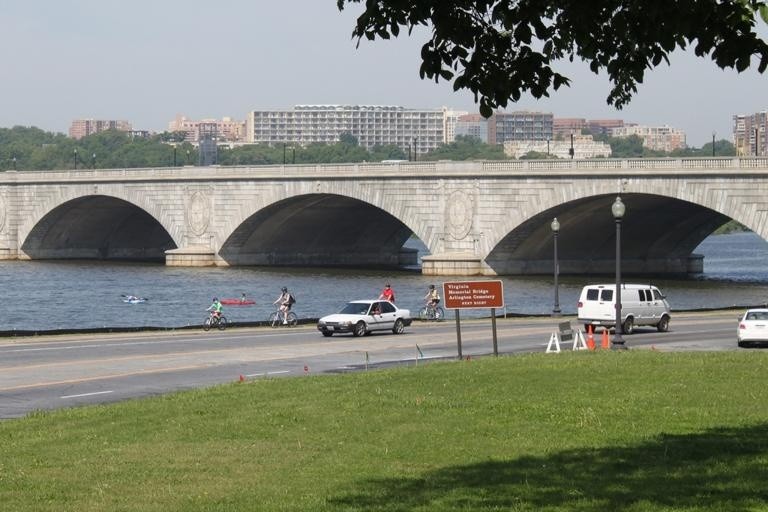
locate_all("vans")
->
[577,282,670,333]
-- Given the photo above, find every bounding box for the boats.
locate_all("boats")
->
[120,296,148,303]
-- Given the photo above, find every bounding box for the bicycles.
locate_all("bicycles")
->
[266,302,297,329]
[417,298,445,322]
[202,309,226,331]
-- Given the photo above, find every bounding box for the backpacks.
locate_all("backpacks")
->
[286,293,296,304]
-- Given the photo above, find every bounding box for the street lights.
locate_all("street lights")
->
[92,151,96,169]
[13,156,16,169]
[283,137,287,163]
[568,127,574,159]
[711,130,716,156]
[292,144,295,164]
[551,217,561,314]
[546,135,550,156]
[173,143,177,166]
[186,149,190,165]
[72,148,77,169]
[610,197,627,352]
[413,134,418,161]
[408,140,412,161]
[752,121,759,167]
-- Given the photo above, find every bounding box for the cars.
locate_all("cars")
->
[734,306,768,348]
[219,297,255,305]
[316,299,411,337]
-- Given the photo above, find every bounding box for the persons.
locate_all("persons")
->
[206,296,222,322]
[421,284,441,320]
[273,285,293,324]
[379,283,393,302]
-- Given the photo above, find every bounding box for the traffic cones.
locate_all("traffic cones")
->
[585,325,594,350]
[600,328,608,350]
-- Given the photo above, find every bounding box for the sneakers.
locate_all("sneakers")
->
[436,312,440,319]
[282,320,288,325]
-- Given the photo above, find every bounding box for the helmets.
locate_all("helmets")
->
[385,284,390,287]
[213,297,218,301]
[281,287,287,291]
[429,285,434,288]
